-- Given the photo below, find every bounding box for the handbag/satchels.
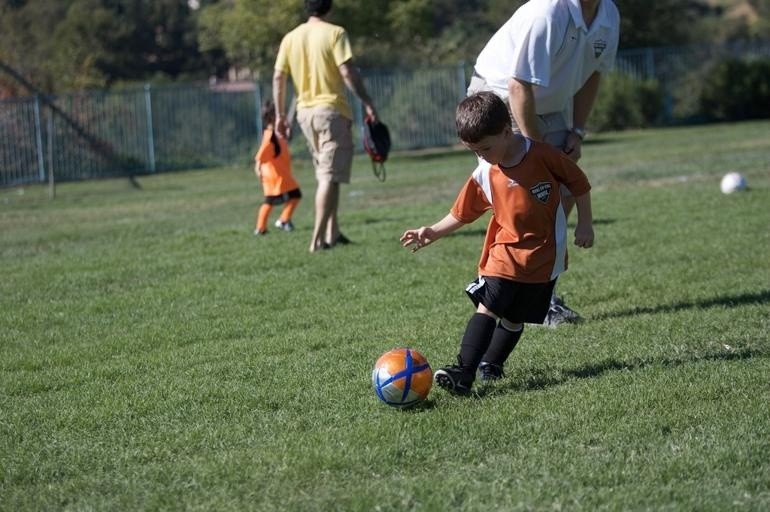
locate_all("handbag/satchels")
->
[362,115,391,163]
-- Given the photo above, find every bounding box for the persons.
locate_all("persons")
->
[274,1,381,254]
[467,1,620,326]
[398,91,595,398]
[253,100,302,236]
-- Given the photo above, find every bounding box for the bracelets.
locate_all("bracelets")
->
[275,112,285,118]
[573,127,587,140]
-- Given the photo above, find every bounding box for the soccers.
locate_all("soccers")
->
[720,173,748,195]
[372,349,433,408]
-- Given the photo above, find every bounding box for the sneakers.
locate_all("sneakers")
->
[433,363,472,396]
[479,361,505,381]
[274,219,296,232]
[531,297,580,329]
[253,228,270,237]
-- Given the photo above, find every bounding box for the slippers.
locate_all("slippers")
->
[308,234,350,254]
